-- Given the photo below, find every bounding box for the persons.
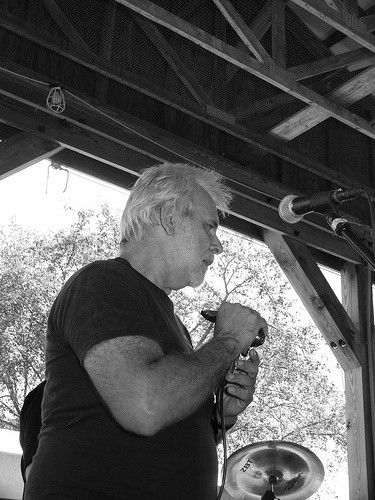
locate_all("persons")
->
[23,163,268,500]
[19,380,46,500]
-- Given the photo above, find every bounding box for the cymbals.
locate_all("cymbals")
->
[221,440,324,500]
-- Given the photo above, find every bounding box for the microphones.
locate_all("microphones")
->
[279,186,365,224]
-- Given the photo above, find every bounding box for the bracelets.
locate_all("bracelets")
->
[213,401,237,431]
